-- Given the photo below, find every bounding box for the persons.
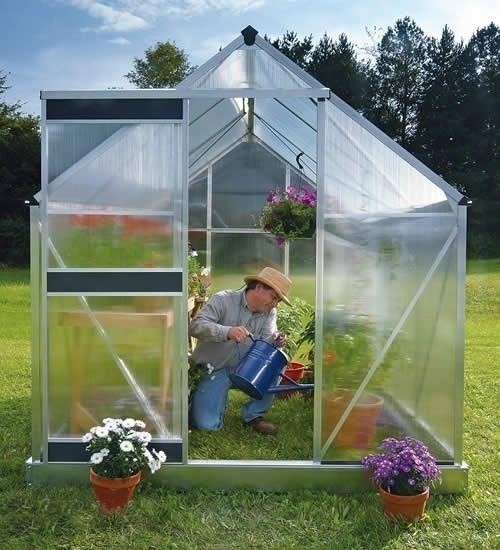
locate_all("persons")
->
[188,266,294,435]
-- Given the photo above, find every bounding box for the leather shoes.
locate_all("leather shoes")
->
[242,416,278,435]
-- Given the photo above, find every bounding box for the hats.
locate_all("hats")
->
[243,266,295,309]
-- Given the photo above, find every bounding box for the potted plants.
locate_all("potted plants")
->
[272,297,409,452]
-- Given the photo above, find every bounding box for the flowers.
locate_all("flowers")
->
[361,434,445,495]
[255,182,319,246]
[82,413,168,480]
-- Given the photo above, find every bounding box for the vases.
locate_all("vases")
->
[284,219,315,241]
[376,483,429,522]
[88,468,142,515]
